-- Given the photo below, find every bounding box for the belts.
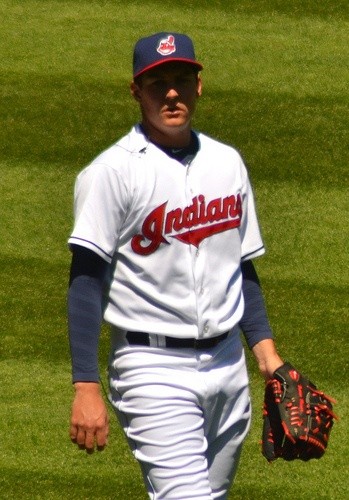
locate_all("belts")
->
[127,328,229,351]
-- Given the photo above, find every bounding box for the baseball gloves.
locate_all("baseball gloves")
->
[259,361,339,463]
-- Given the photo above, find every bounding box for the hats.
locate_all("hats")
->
[132,32,202,77]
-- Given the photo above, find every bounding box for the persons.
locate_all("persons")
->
[64,32,292,500]
